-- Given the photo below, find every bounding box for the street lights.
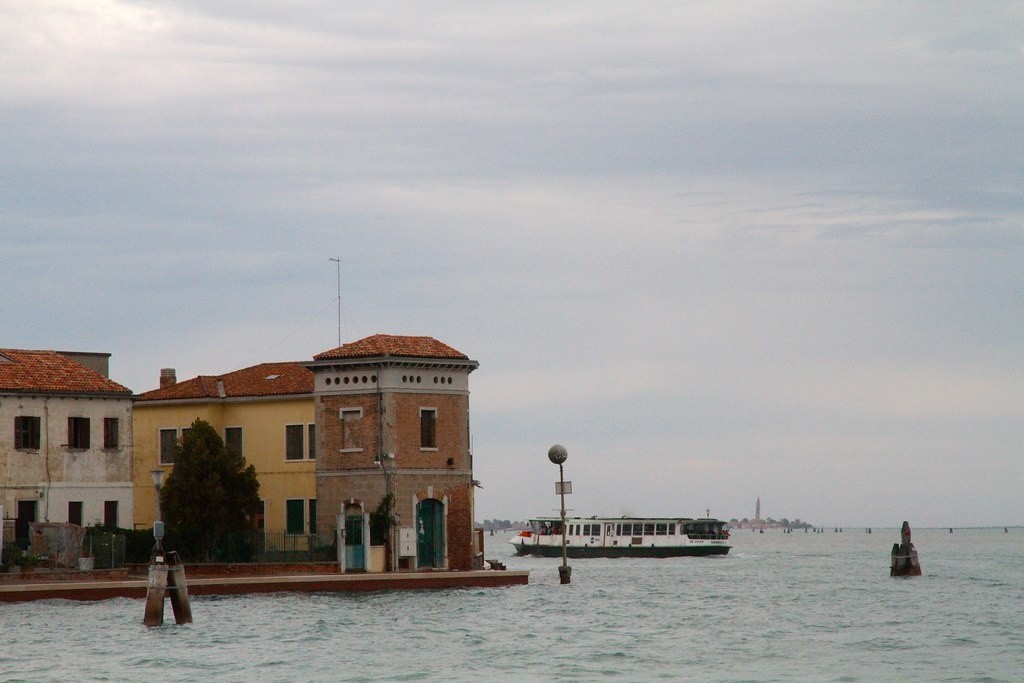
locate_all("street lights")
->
[150,467,165,563]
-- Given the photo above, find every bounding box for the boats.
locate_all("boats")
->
[508,516,734,556]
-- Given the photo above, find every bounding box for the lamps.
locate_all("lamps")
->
[373,454,383,469]
